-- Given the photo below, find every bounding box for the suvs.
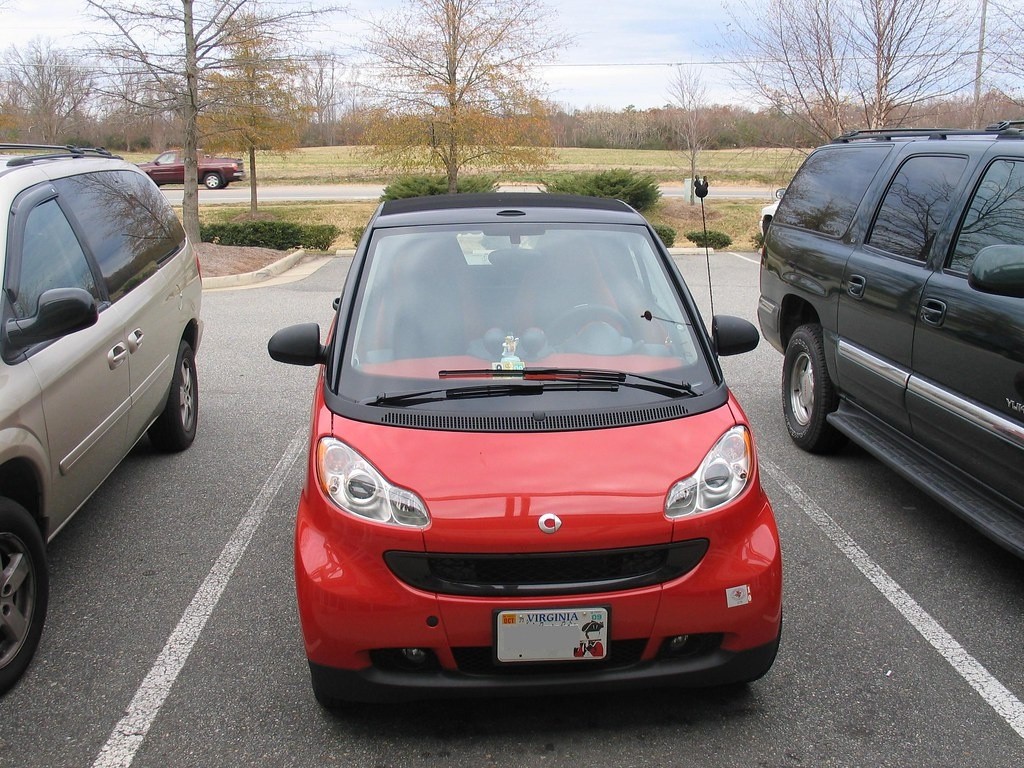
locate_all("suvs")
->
[0,142,204,696]
[759,121,1023,563]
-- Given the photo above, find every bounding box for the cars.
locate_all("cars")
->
[760,188,786,237]
[267,193,784,717]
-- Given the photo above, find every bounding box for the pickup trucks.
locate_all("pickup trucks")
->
[137,149,246,190]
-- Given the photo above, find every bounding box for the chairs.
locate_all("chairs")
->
[510,231,622,334]
[374,241,487,359]
